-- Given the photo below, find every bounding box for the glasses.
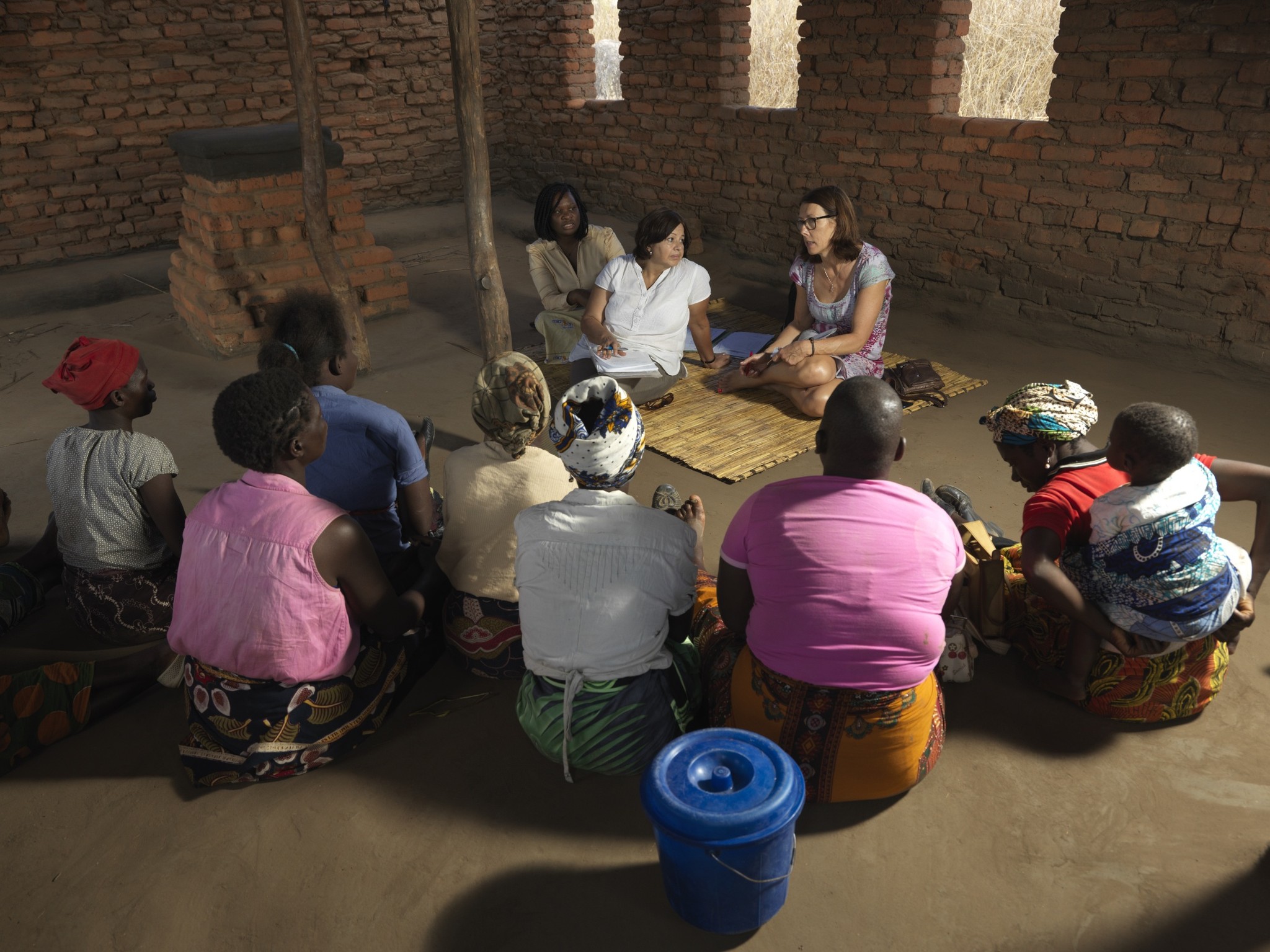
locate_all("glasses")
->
[645,393,673,409]
[795,215,833,231]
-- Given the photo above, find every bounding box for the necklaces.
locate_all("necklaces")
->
[823,265,840,292]
[563,245,577,256]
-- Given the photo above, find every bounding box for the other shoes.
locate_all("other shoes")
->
[651,484,683,516]
[420,416,435,482]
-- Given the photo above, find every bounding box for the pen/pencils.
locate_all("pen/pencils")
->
[604,346,628,351]
[746,351,753,376]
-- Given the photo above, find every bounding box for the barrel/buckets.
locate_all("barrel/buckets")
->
[640,727,807,937]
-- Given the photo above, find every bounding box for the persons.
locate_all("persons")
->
[258,286,445,589]
[513,375,703,783]
[431,352,563,680]
[675,377,967,804]
[0,335,186,774]
[525,183,627,365]
[158,368,443,786]
[920,379,1255,701]
[716,188,896,417]
[568,206,732,404]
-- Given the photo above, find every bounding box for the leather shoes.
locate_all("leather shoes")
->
[935,485,1004,539]
[921,478,956,517]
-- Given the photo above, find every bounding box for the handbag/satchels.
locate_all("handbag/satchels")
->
[938,615,1011,684]
[942,520,1004,636]
[881,359,947,407]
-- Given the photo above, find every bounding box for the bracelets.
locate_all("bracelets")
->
[809,337,814,357]
